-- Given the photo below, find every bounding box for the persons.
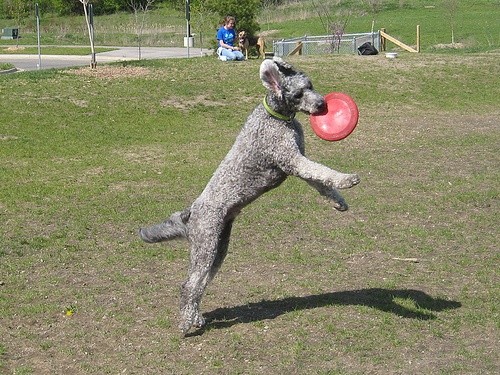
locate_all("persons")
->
[217,16,244,61]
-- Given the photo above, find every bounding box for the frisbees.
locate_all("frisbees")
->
[310,92,359,142]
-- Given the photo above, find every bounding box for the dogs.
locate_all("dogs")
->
[140,57,361,337]
